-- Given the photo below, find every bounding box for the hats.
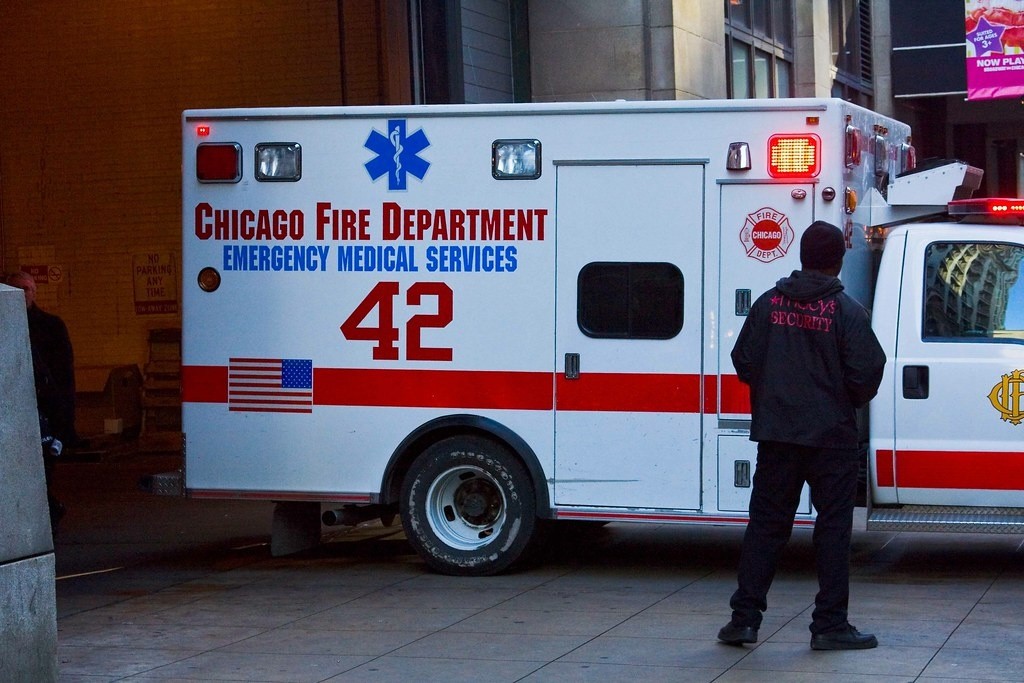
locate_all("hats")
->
[800,221,846,268]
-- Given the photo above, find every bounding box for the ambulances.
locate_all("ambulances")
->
[140,98,1024,579]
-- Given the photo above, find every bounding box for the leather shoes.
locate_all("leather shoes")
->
[811,627,880,650]
[716,622,759,643]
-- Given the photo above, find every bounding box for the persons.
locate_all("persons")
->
[6,271,77,529]
[711,218,888,654]
